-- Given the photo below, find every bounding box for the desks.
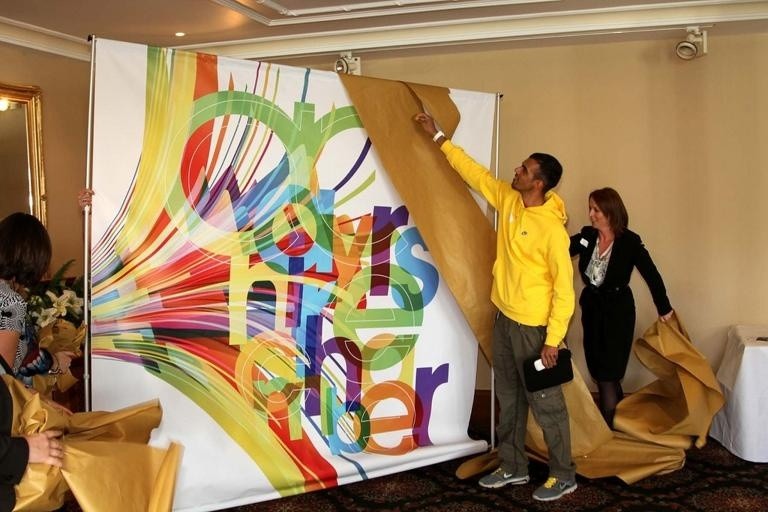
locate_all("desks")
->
[700,329,767,464]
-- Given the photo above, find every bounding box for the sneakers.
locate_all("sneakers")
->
[531,474,580,501]
[478,465,531,489]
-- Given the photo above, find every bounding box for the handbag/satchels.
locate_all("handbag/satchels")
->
[522,348,574,392]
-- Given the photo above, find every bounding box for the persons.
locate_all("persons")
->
[0,369,66,512]
[410,111,578,501]
[77,187,95,208]
[568,185,674,429]
[1,211,84,375]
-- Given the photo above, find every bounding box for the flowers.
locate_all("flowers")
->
[21,256,91,334]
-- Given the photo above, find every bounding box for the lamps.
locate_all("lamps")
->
[671,24,711,61]
[333,48,362,80]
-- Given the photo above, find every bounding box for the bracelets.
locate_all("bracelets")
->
[433,131,446,143]
